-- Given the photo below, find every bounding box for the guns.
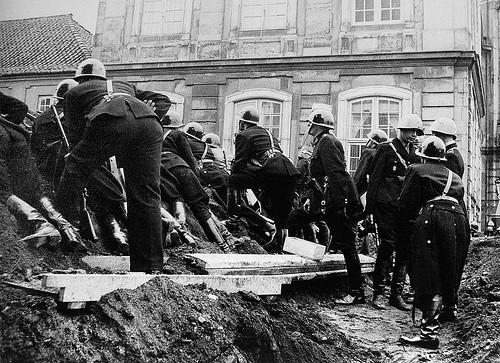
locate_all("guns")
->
[52,106,97,244]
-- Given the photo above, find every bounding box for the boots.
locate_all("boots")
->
[444,303,458,320]
[172,201,186,245]
[103,214,129,252]
[389,258,411,311]
[277,229,288,254]
[6,194,62,247]
[399,300,443,349]
[39,196,88,254]
[371,254,392,310]
[199,217,233,253]
[209,210,245,249]
[160,208,197,248]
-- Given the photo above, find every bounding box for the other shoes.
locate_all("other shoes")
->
[264,221,276,246]
[335,285,365,304]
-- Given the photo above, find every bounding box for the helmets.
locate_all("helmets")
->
[52,79,79,99]
[184,122,204,139]
[237,106,260,124]
[396,113,424,135]
[201,133,220,148]
[415,136,448,161]
[428,118,457,140]
[366,129,388,144]
[306,109,335,130]
[73,59,108,80]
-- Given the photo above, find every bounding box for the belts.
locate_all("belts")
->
[425,195,459,204]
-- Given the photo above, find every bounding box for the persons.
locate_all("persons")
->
[1,92,88,251]
[31,79,129,256]
[181,123,278,249]
[414,117,464,328]
[383,132,424,302]
[352,128,387,256]
[53,58,170,275]
[201,132,276,246]
[372,115,423,310]
[220,107,299,254]
[158,111,231,253]
[399,136,471,348]
[302,109,365,304]
